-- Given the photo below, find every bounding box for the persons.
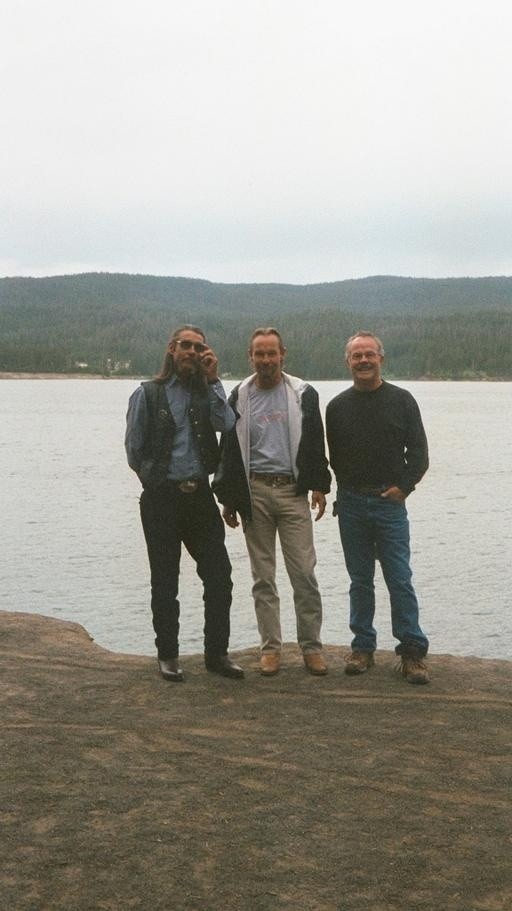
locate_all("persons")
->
[208,327,332,674]
[324,332,431,685]
[125,326,247,679]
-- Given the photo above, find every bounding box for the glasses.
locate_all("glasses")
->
[176,340,204,352]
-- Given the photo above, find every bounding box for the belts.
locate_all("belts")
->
[178,481,198,493]
[263,474,289,487]
[354,482,384,497]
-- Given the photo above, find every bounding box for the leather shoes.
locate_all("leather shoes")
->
[206,656,243,678]
[158,658,184,681]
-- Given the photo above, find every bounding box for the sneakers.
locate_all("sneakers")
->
[344,652,374,674]
[303,653,328,675]
[260,654,280,676]
[395,655,429,683]
